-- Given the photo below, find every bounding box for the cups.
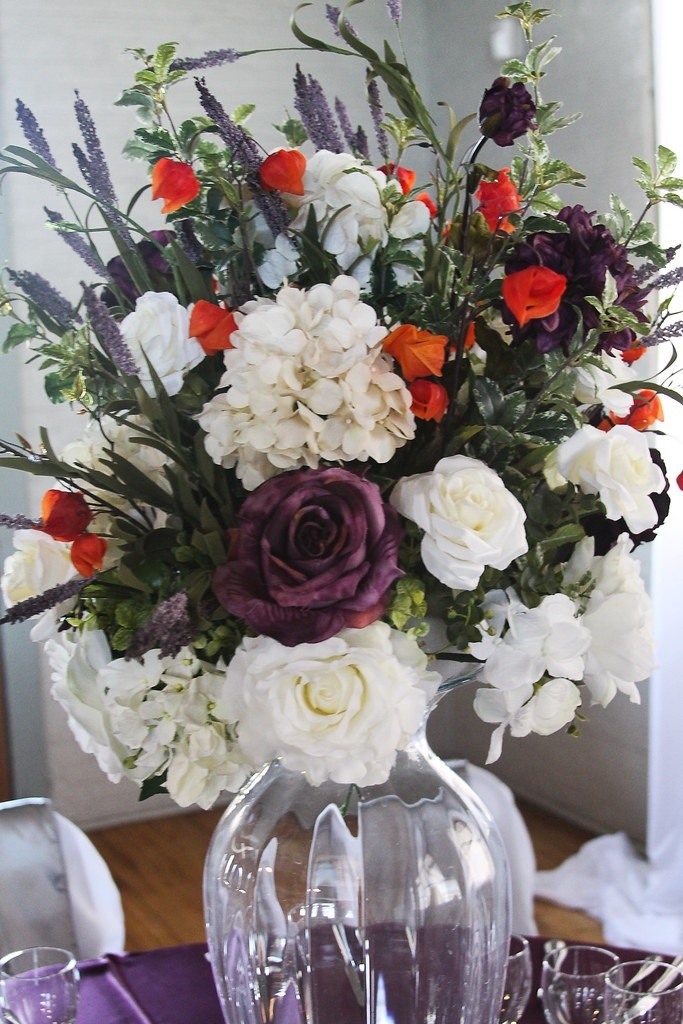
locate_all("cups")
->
[500,932,533,1023]
[1,945,81,1023]
[604,959,682,1023]
[540,945,624,1024]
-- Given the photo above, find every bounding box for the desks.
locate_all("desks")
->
[0,924,683,1024]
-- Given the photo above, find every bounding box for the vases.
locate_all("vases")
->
[203,688,513,1024]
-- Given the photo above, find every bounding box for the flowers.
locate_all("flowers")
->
[1,0,683,808]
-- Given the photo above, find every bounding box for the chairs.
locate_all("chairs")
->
[0,796,127,979]
